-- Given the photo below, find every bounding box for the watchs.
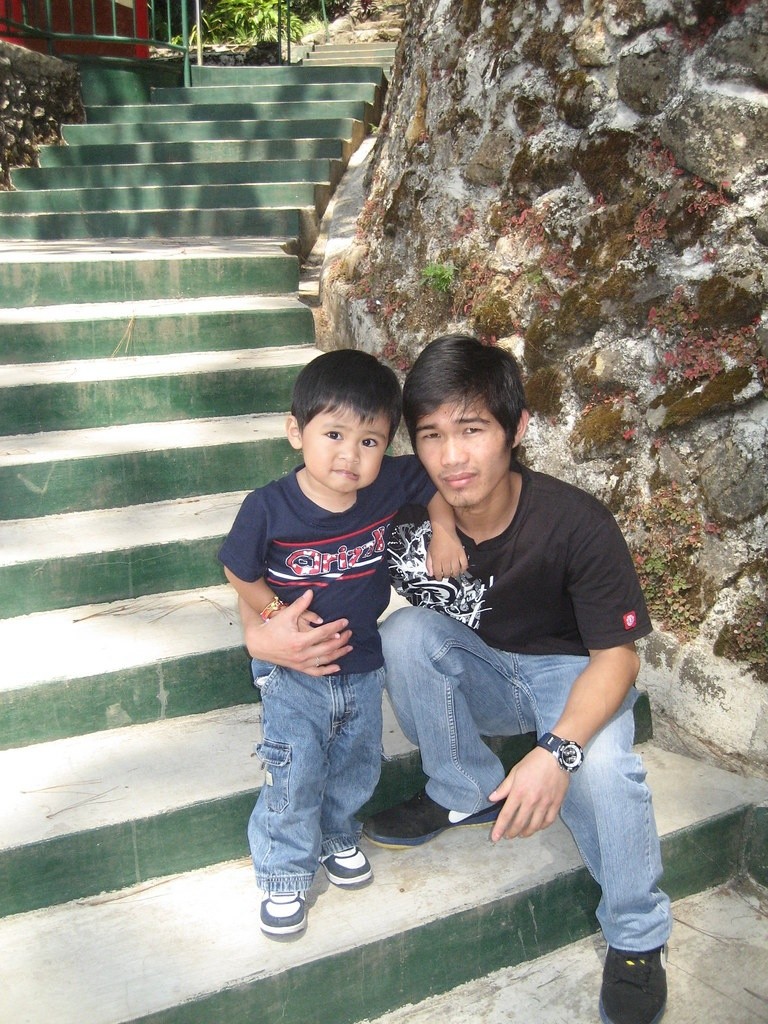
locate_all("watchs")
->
[535,732,584,772]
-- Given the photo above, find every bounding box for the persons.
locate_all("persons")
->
[361,331,670,1024]
[219,349,470,936]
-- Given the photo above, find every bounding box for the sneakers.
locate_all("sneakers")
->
[600,940,668,1024]
[317,846,372,886]
[260,889,306,935]
[363,788,508,850]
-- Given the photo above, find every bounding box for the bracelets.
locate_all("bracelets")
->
[257,595,288,621]
